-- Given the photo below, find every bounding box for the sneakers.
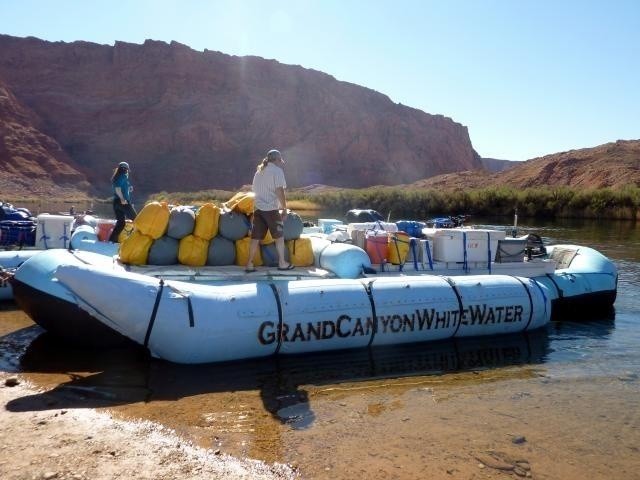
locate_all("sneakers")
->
[107,240,120,244]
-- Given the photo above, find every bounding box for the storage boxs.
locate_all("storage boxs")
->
[495,238,529,263]
[422,227,506,262]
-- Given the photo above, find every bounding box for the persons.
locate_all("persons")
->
[243,149,295,273]
[69,210,102,250]
[107,161,137,244]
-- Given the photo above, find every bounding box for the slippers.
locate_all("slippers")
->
[277,264,295,270]
[245,267,256,272]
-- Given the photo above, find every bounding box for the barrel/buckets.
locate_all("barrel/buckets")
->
[388,231,410,265]
[365,230,389,264]
[97,219,115,238]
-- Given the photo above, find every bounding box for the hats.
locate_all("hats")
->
[267,150,284,166]
[120,162,129,169]
